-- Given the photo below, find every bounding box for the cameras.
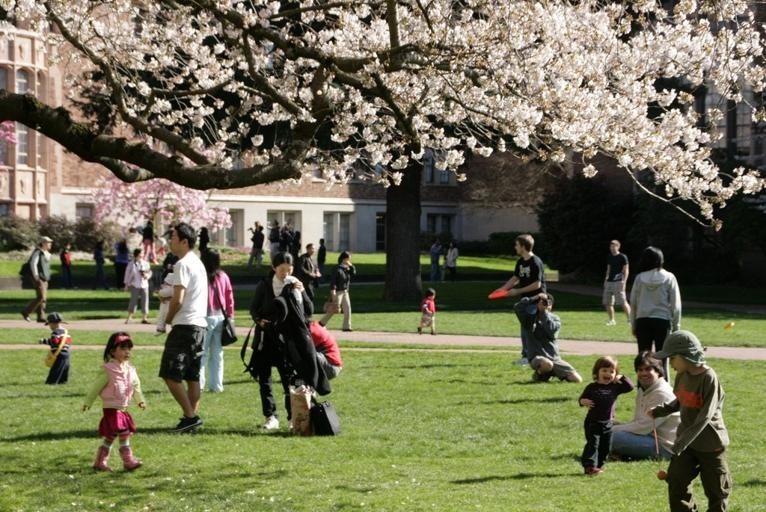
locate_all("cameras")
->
[542,299,552,306]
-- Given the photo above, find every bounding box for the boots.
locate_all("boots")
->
[92,444,115,472]
[118,444,144,472]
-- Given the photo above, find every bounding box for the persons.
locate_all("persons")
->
[647,330,732,510]
[84,333,146,473]
[445,241,459,282]
[577,355,633,475]
[418,288,437,335]
[95,220,210,335]
[320,250,356,331]
[500,232,545,363]
[61,241,78,289]
[247,219,326,303]
[201,247,233,393]
[430,238,444,280]
[306,320,341,380]
[610,350,683,462]
[38,314,71,384]
[515,290,582,383]
[21,235,53,323]
[601,240,633,326]
[250,251,314,428]
[629,246,682,382]
[159,221,208,432]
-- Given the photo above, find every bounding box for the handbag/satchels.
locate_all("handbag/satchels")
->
[221,316,239,347]
[309,400,343,437]
[44,350,58,368]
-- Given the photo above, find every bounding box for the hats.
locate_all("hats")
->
[650,328,706,360]
[44,312,63,326]
[37,236,54,245]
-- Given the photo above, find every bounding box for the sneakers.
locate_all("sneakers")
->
[583,462,606,477]
[257,414,293,431]
[602,318,634,327]
[511,356,573,383]
[417,326,439,336]
[123,318,155,325]
[318,321,354,332]
[165,413,204,435]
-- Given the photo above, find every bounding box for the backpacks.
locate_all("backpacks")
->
[18,260,39,291]
[241,318,290,379]
